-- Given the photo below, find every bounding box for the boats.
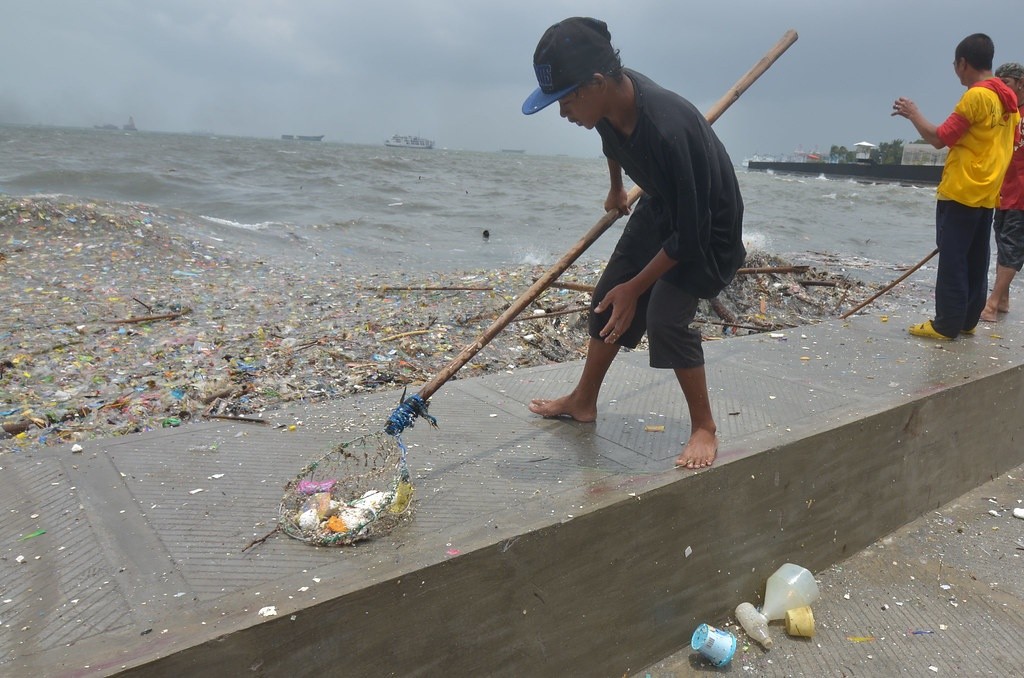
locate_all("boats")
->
[94,124,118,129]
[501,149,527,153]
[385,134,435,149]
[123,117,138,131]
[282,134,325,141]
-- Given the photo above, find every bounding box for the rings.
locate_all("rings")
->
[895,105,898,108]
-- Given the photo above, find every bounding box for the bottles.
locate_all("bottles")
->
[759,563,819,625]
[735,602,773,649]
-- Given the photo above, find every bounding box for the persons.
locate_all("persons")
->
[976,63,1024,322]
[891,33,1021,340]
[521,16,748,469]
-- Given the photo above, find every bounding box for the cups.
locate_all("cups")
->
[692,623,736,667]
[785,606,815,637]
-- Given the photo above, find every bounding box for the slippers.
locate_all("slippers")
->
[958,327,976,334]
[908,319,957,341]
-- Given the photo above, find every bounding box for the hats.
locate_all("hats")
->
[995,62,1024,79]
[522,17,614,115]
